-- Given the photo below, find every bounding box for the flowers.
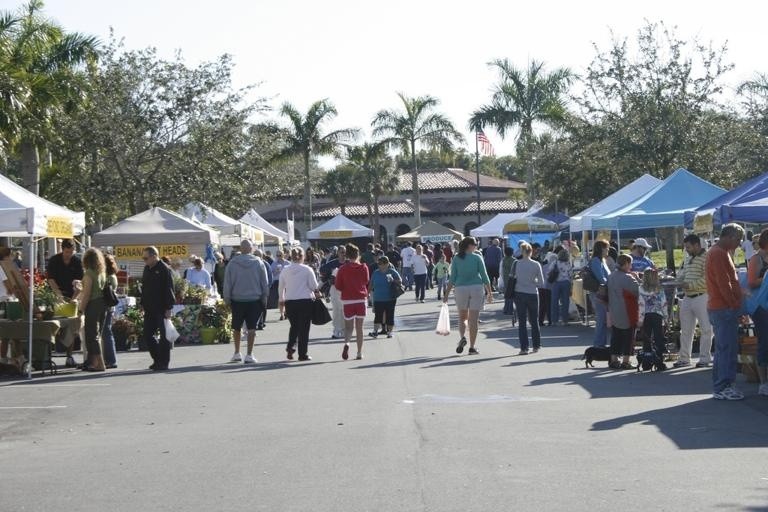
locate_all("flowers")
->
[21,266,50,285]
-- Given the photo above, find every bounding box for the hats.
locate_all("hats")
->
[62,239,74,248]
[634,239,652,248]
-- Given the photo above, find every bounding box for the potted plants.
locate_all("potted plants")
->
[7,287,80,321]
[115,274,234,350]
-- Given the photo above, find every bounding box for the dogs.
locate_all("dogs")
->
[581,342,625,369]
[636,349,667,372]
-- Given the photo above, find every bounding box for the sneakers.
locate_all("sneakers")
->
[231,352,241,360]
[65,356,117,370]
[611,361,636,369]
[333,331,344,338]
[0,357,32,375]
[456,337,466,353]
[758,384,768,395]
[674,361,689,368]
[696,362,708,367]
[369,330,393,338]
[149,362,168,370]
[299,354,311,360]
[245,355,256,362]
[357,352,363,359]
[286,349,295,360]
[342,343,349,360]
[469,348,479,355]
[713,386,744,400]
[519,350,527,355]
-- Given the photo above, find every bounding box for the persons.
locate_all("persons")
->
[586,239,612,348]
[278,246,319,361]
[100,254,118,369]
[0,246,28,362]
[704,222,747,401]
[672,234,713,368]
[508,242,545,356]
[637,267,669,371]
[46,238,84,368]
[161,231,768,340]
[334,242,371,361]
[746,230,768,396]
[11,251,24,269]
[440,236,493,355]
[77,247,108,372]
[607,254,640,371]
[223,239,268,364]
[140,245,176,371]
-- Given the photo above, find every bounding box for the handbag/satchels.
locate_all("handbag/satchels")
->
[583,272,599,291]
[104,285,119,306]
[312,299,331,325]
[547,265,559,283]
[391,281,404,298]
[505,278,515,298]
[736,271,768,317]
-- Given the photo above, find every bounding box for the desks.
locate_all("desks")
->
[0,316,82,376]
[572,278,592,327]
